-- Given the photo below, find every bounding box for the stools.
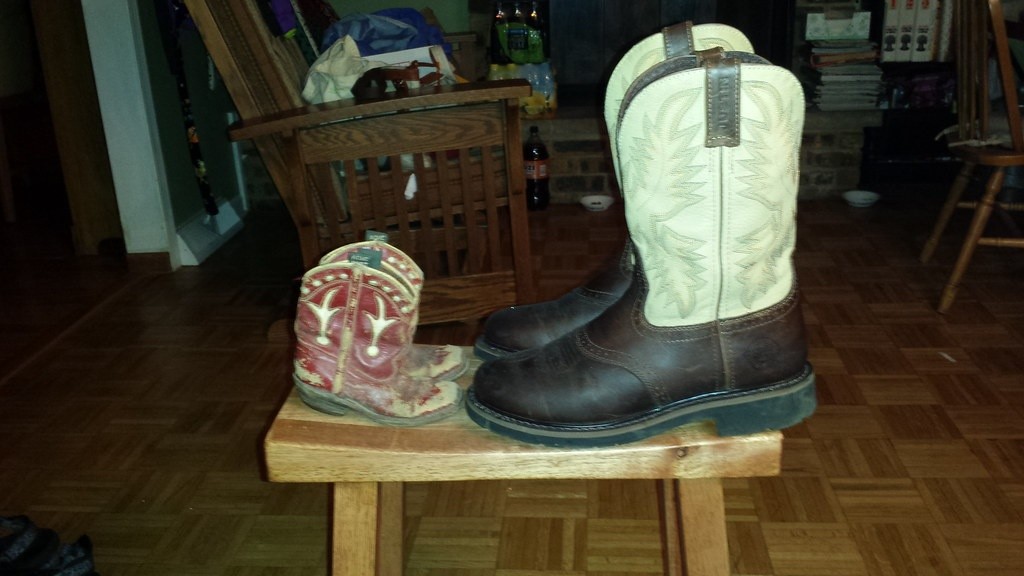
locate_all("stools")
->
[263,348,783,576]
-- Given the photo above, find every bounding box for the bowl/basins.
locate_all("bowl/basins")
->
[580,195,614,211]
[842,190,880,207]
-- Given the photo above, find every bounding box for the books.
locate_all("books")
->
[808,40,883,111]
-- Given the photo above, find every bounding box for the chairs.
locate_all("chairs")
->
[185,0,543,347]
[920,0,1024,314]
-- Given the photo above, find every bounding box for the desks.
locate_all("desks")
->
[514,109,884,205]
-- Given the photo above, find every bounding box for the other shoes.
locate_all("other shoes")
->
[0,516,100,576]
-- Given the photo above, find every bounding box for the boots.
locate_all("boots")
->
[466,50,816,445]
[291,259,462,425]
[319,240,468,381]
[472,21,753,360]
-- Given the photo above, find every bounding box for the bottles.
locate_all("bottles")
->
[486,0,557,121]
[522,126,550,212]
